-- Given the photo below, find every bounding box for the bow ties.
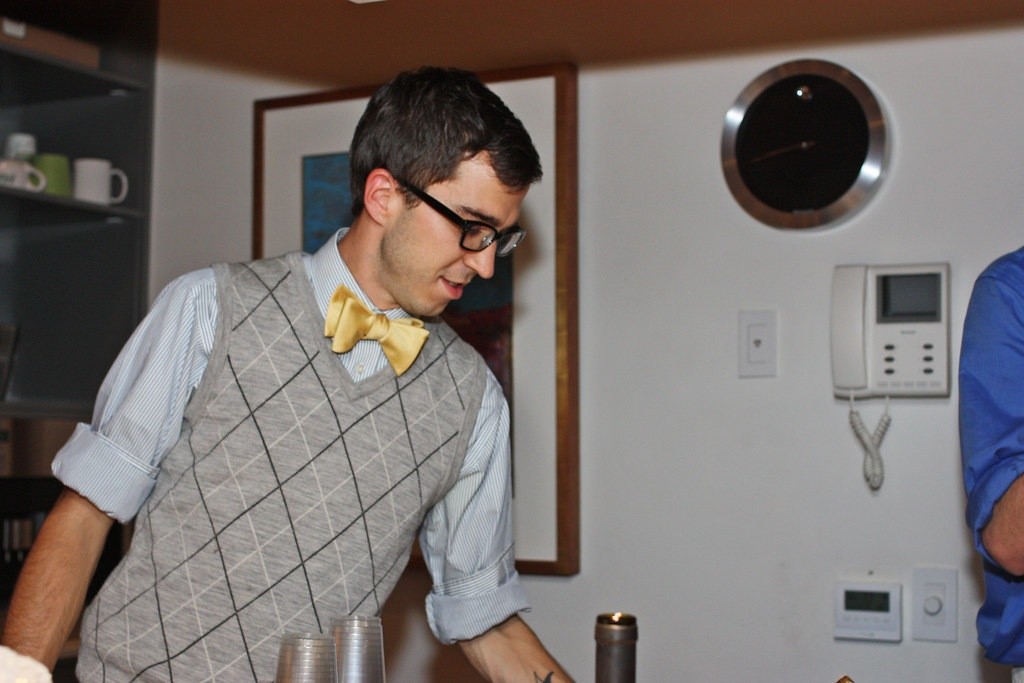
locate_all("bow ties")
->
[323,284,431,377]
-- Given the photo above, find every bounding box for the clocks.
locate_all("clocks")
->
[721,58,893,230]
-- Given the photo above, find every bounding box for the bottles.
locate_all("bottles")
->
[593,613,640,683]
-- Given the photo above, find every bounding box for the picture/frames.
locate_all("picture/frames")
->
[251,62,583,577]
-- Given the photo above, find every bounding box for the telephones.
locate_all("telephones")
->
[832,262,955,401]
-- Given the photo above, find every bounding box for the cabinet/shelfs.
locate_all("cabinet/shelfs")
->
[2,0,161,683]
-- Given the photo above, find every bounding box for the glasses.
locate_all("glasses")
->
[390,175,527,257]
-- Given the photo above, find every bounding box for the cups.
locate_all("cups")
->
[0,159,47,194]
[71,157,129,207]
[275,614,386,683]
[35,152,72,199]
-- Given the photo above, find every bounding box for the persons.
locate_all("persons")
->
[0,65,578,682]
[957,244,1024,682]
